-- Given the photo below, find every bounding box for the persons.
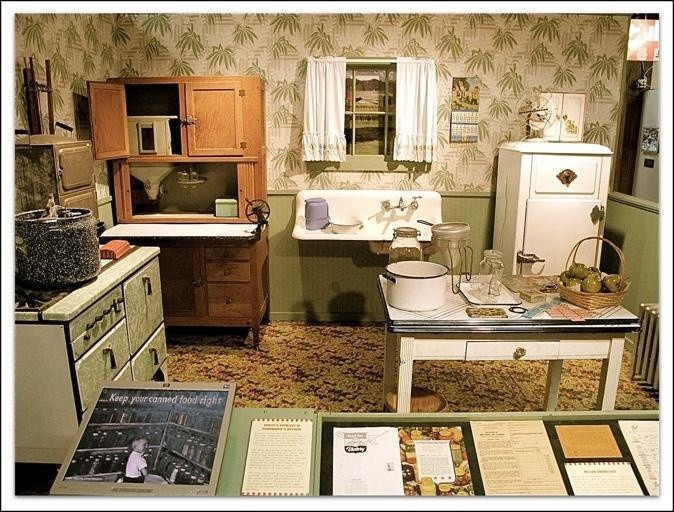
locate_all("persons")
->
[123,436,148,483]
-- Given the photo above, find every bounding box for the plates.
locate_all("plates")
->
[456,282,522,305]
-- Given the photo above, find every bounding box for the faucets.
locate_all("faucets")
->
[399,196,404,212]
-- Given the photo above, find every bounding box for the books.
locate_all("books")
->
[564,461,645,495]
[470,420,569,496]
[332,426,475,495]
[241,418,314,496]
[555,424,624,458]
[618,420,661,495]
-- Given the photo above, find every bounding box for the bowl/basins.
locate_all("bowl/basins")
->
[327,217,362,234]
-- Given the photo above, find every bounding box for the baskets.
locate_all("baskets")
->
[557,237,631,311]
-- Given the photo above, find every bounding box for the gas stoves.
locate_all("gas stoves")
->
[14,286,72,322]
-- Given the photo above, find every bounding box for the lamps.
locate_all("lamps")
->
[625,19,659,88]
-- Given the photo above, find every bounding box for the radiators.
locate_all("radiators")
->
[629,302,659,390]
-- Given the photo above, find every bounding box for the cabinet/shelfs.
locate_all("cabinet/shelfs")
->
[15,247,169,464]
[84,78,271,348]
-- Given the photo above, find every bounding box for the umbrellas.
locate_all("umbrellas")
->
[466,307,508,319]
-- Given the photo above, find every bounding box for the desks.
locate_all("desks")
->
[49,409,661,498]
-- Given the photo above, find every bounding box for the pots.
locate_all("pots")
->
[382,260,447,312]
[16,206,102,286]
